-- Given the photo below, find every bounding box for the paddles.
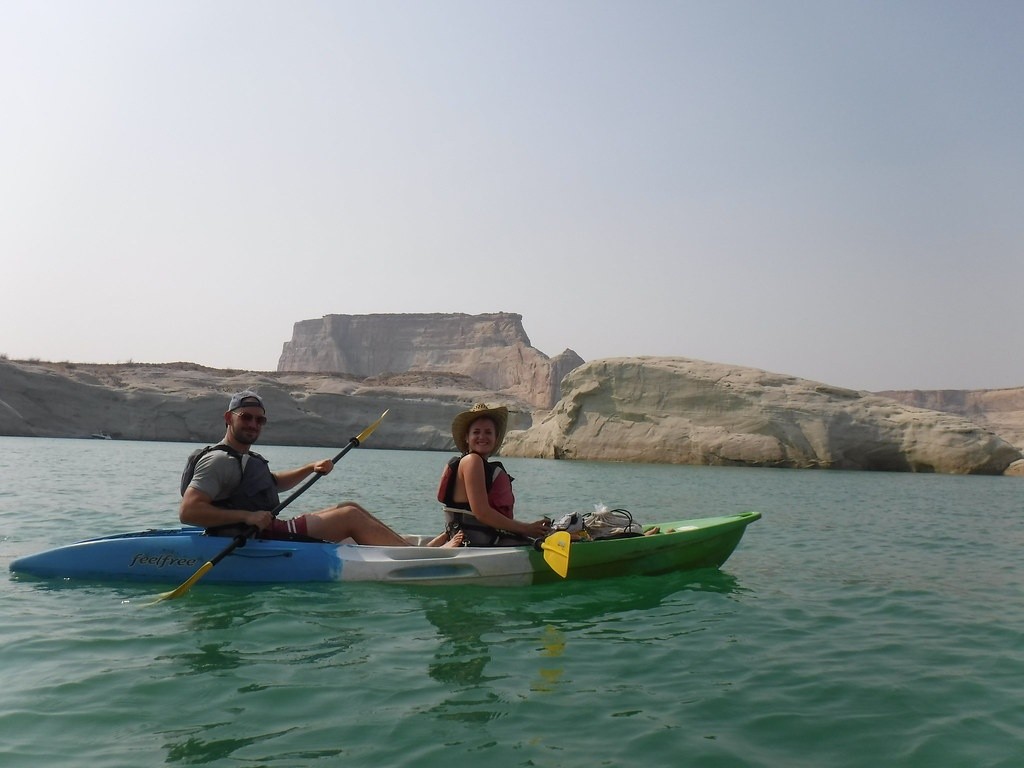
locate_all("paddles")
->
[163,405,391,601]
[503,528,572,578]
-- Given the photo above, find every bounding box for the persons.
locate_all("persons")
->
[179,391,464,548]
[437,403,659,547]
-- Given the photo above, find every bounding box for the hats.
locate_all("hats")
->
[225,390,266,429]
[452,402,509,458]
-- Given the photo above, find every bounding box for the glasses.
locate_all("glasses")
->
[231,411,267,425]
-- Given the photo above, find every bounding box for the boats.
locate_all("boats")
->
[6,512,762,585]
